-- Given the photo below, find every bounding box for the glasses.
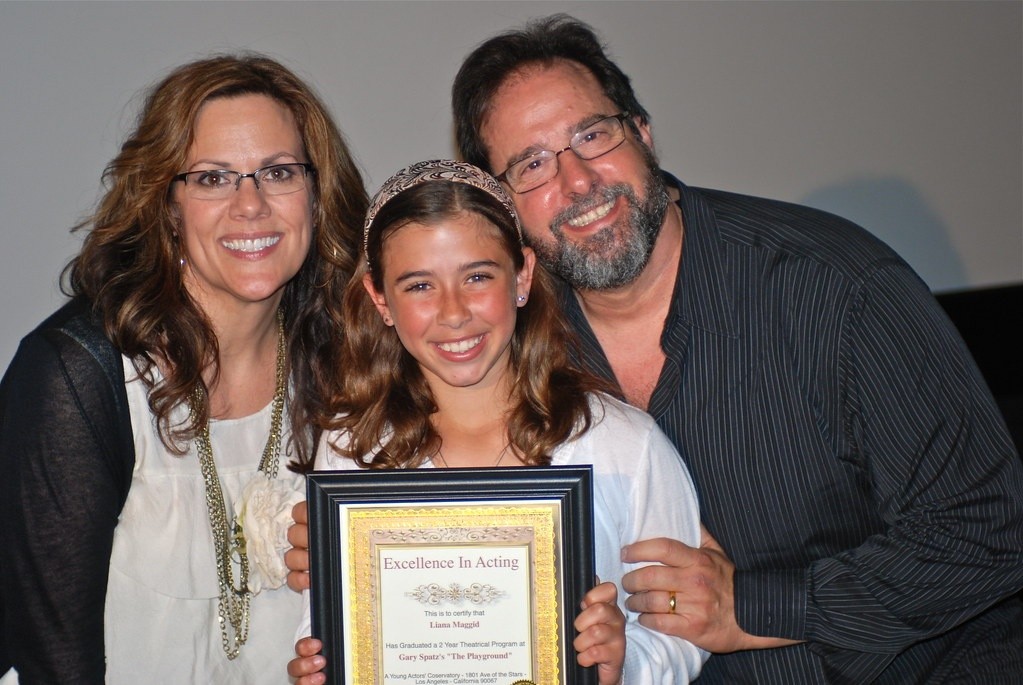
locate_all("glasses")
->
[171,161,314,199]
[492,110,636,194]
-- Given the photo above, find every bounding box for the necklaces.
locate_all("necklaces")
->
[179,309,293,655]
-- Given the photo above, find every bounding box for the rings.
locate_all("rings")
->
[668,590,677,614]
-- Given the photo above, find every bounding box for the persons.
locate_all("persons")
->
[292,155,712,684]
[284,14,1023,684]
[0,50,374,684]
